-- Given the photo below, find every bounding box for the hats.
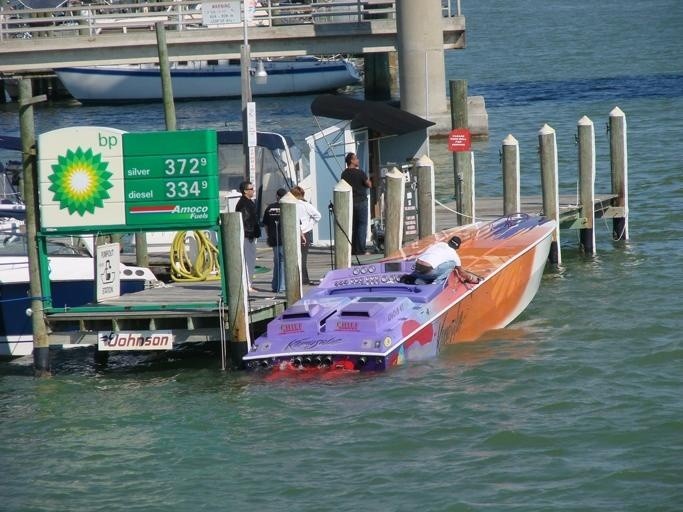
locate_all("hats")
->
[449,236,461,249]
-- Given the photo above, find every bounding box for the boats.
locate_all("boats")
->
[242,215,556,369]
[0,203,165,358]
[216,125,310,227]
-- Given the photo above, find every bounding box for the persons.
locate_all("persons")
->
[290,185,322,286]
[339,152,374,258]
[413,234,481,285]
[234,181,264,293]
[261,187,308,294]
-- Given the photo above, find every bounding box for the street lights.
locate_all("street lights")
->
[240,43,268,199]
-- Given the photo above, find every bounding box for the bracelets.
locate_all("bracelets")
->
[469,274,472,282]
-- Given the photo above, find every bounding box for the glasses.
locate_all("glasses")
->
[245,188,253,190]
